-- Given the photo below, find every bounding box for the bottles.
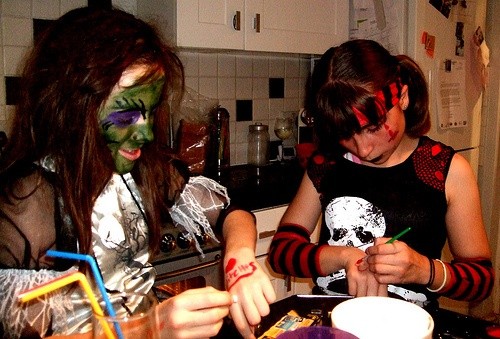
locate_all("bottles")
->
[208,106,232,187]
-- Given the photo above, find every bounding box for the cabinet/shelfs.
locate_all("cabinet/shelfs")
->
[111,0,351,58]
[250,204,328,301]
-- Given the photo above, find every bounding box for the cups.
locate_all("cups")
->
[87,291,160,339]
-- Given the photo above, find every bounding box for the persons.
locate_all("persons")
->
[270,38,495,304]
[1,5,276,339]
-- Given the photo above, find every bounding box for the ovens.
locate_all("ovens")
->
[147,223,224,308]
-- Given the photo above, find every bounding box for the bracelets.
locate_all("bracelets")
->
[426,258,447,292]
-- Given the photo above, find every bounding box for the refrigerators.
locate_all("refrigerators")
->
[347,0,488,191]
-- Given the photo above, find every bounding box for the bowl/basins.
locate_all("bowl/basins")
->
[331,296,435,339]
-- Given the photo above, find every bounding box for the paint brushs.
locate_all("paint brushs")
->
[356,228,411,264]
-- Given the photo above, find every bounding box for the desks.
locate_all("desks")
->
[189,296,500,339]
